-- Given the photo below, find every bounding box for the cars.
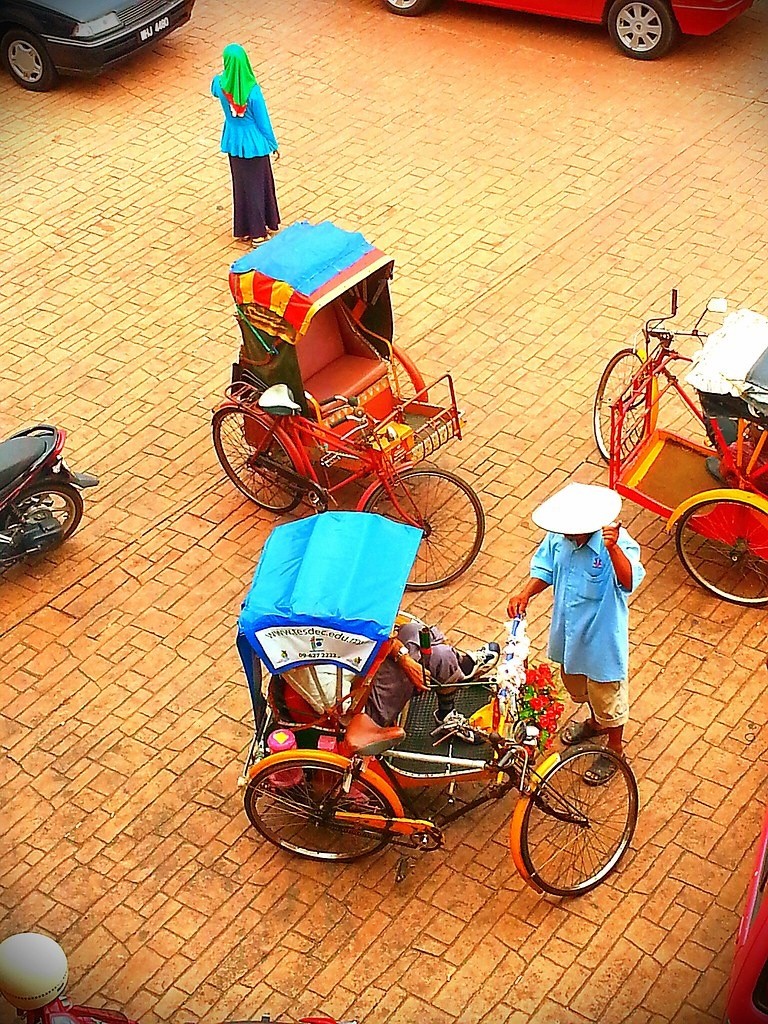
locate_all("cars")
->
[0,0,197,93]
[384,1,752,61]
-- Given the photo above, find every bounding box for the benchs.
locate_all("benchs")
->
[266,664,356,733]
[295,300,392,423]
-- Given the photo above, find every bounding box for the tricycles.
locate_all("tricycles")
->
[593,287,768,607]
[209,221,486,593]
[234,511,638,901]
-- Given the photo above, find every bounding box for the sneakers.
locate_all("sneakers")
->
[433,708,487,744]
[457,642,501,689]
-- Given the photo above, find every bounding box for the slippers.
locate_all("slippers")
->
[583,752,625,786]
[251,233,272,248]
[238,234,248,241]
[560,718,609,745]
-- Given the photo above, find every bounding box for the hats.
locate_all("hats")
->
[531,482,623,535]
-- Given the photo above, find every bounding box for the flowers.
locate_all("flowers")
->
[520,664,564,756]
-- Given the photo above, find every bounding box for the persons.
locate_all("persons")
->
[507,480,646,785]
[705,415,738,488]
[211,44,282,247]
[287,622,501,745]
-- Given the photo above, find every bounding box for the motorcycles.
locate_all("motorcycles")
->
[1,423,98,582]
